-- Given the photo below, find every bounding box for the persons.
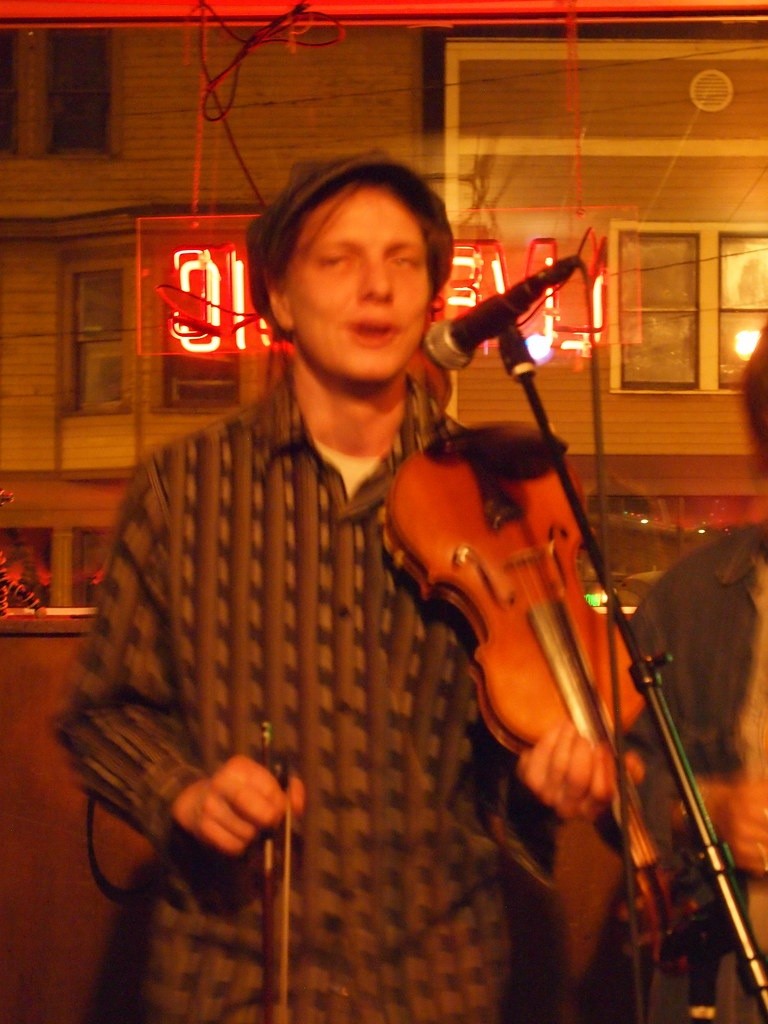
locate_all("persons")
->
[50,145,650,1024]
[625,325,768,1024]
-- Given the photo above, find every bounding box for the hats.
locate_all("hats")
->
[242,146,454,316]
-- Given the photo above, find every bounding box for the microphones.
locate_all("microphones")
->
[421,254,580,372]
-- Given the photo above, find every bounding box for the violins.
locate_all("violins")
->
[377,418,713,975]
